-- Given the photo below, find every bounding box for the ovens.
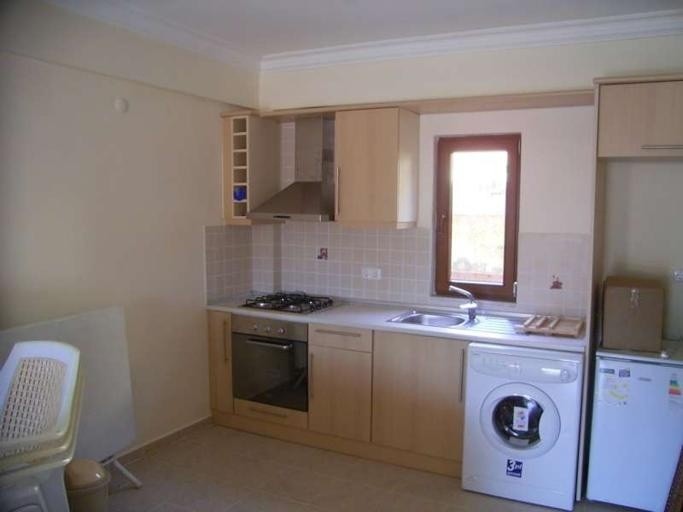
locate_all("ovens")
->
[231,333,308,414]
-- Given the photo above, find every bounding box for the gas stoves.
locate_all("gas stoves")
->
[230,289,348,342]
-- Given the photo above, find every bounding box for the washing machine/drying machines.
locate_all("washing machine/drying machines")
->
[460,340,584,511]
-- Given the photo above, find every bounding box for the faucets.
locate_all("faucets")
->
[448,285,477,323]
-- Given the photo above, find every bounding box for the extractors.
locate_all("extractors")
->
[243,111,335,222]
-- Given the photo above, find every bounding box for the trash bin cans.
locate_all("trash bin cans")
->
[63,459,112,512]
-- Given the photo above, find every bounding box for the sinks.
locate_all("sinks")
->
[386,310,478,329]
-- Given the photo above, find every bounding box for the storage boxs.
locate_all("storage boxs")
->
[599,273,670,355]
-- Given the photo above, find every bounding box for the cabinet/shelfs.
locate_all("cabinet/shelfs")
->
[221,108,280,230]
[205,308,232,426]
[228,396,309,449]
[593,74,682,164]
[305,322,372,459]
[331,107,420,230]
[373,326,469,478]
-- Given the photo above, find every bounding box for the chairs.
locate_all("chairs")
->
[1,338,85,512]
